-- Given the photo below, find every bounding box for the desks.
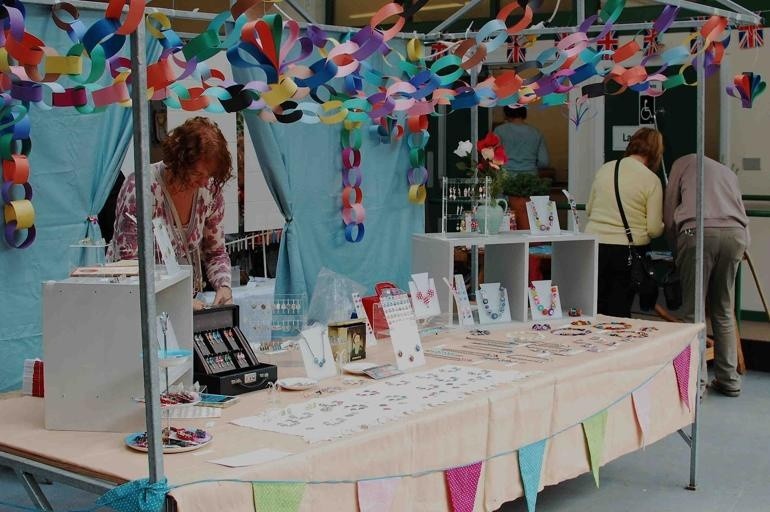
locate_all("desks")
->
[0,314,707,512]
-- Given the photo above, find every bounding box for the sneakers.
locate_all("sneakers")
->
[706,374,740,398]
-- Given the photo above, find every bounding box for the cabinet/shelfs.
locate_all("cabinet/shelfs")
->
[42,264,195,431]
[413,229,599,329]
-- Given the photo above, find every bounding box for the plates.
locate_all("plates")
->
[277,377,320,391]
[343,362,377,375]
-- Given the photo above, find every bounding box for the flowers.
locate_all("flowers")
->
[453,133,509,197]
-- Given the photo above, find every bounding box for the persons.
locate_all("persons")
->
[582,126,664,320]
[103,115,233,313]
[493,102,552,176]
[349,333,366,362]
[663,153,748,397]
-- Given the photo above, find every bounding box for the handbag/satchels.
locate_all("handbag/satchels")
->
[624,249,674,289]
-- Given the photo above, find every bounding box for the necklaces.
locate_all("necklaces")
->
[304,331,327,367]
[412,276,436,310]
[530,285,558,316]
[479,287,506,320]
[529,201,555,231]
[424,318,659,366]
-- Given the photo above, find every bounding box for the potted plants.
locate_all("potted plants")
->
[503,173,552,230]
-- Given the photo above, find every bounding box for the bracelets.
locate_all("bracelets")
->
[131,389,206,449]
